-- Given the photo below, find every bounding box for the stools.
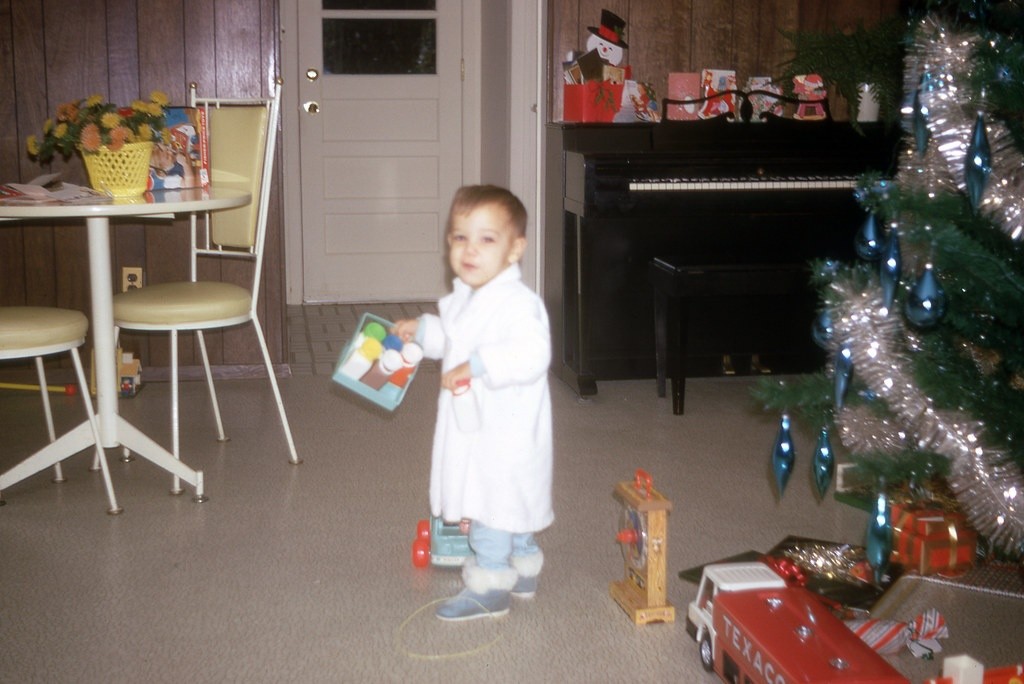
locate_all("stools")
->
[640,246,829,414]
[1,308,125,518]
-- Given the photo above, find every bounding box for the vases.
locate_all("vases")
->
[79,143,152,197]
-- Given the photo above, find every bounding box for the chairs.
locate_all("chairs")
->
[110,77,303,496]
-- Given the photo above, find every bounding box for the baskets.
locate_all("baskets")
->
[79,140,154,197]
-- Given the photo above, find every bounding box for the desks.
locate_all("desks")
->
[0,185,252,504]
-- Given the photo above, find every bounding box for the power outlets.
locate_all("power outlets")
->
[122,267,142,292]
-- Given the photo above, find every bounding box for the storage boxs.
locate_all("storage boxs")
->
[564,82,653,123]
[890,503,976,575]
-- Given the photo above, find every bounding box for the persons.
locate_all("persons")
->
[390,185,554,620]
[149,147,195,188]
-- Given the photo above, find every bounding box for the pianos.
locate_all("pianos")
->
[543,119,914,401]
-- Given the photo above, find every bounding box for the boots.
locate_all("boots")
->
[511,551,543,597]
[434,568,517,621]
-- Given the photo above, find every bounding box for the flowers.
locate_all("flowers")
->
[26,90,172,165]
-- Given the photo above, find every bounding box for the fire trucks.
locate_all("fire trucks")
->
[684,557,912,684]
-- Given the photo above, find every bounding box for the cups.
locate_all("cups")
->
[856,82,880,121]
